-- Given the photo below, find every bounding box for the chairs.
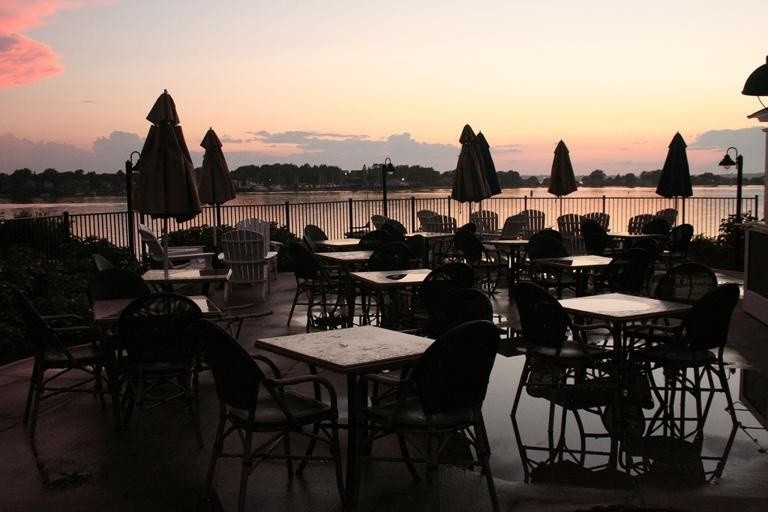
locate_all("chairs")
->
[196,316,344,509]
[12,283,121,437]
[641,262,736,465]
[110,292,207,430]
[511,280,630,481]
[624,284,740,488]
[87,198,754,384]
[350,320,500,512]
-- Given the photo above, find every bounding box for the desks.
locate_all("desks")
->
[256,324,449,511]
[552,288,701,475]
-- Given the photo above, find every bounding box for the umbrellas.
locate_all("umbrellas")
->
[197,125,236,229]
[473,129,501,231]
[653,130,693,231]
[129,87,204,281]
[547,139,578,237]
[447,123,491,230]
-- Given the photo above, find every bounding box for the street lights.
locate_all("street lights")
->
[381,157,395,216]
[124,148,140,254]
[718,146,744,222]
